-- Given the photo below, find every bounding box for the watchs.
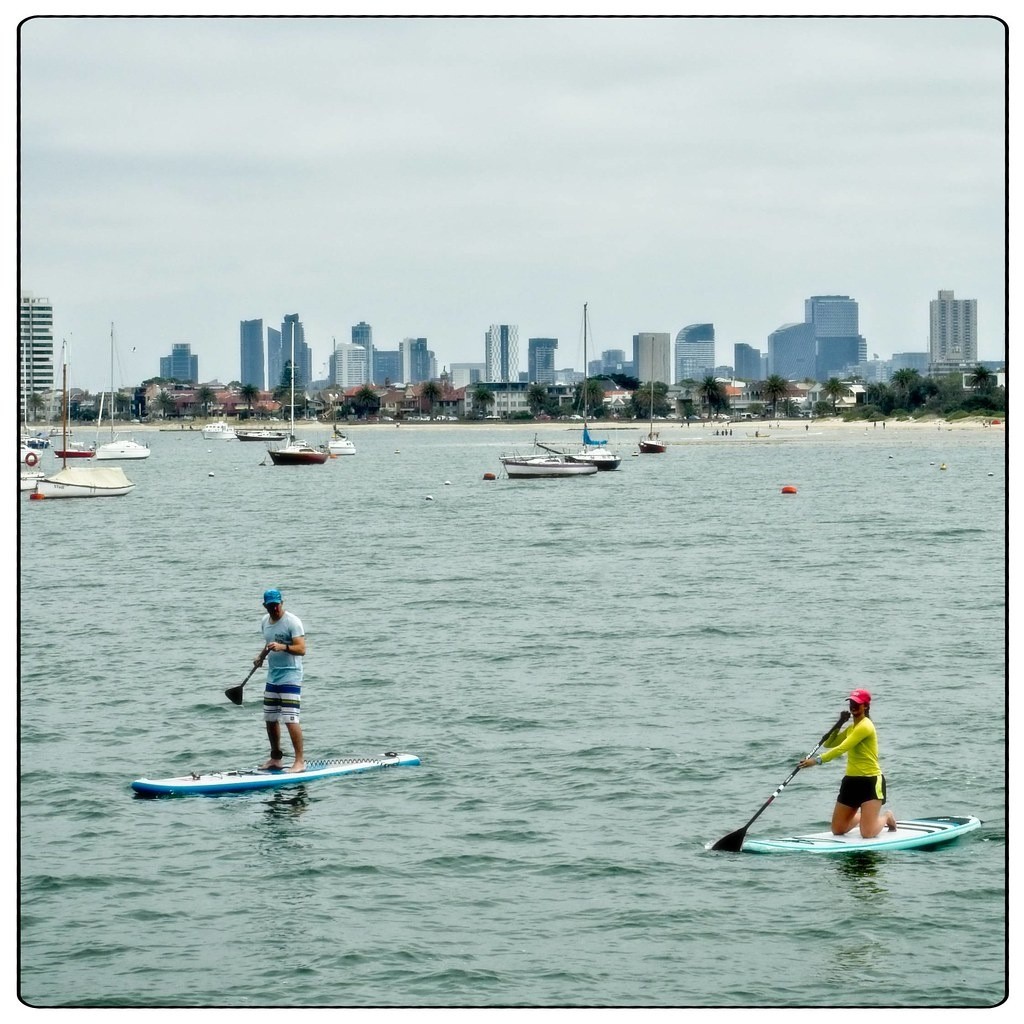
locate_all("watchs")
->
[815,756,823,766]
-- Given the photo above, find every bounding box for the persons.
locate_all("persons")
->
[252,588,306,774]
[794,687,898,839]
[680,420,810,438]
[862,420,888,432]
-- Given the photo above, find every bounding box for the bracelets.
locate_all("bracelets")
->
[284,644,290,651]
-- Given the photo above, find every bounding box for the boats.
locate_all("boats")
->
[746,430,771,437]
[326,427,356,457]
[19,424,54,465]
[499,452,600,479]
[235,425,286,442]
[21,464,45,491]
[48,427,75,437]
[200,419,237,439]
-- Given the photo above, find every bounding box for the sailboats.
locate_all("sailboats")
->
[636,336,667,452]
[29,337,137,502]
[265,319,329,467]
[52,370,97,459]
[92,321,152,461]
[535,300,623,472]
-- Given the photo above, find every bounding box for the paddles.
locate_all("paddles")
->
[712,712,847,851]
[225,645,270,705]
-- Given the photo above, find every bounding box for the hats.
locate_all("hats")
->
[263,589,282,605]
[845,688,871,705]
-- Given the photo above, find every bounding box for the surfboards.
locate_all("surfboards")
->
[131,751,420,793]
[742,814,981,852]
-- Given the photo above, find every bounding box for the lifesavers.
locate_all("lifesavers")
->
[25,452,38,467]
